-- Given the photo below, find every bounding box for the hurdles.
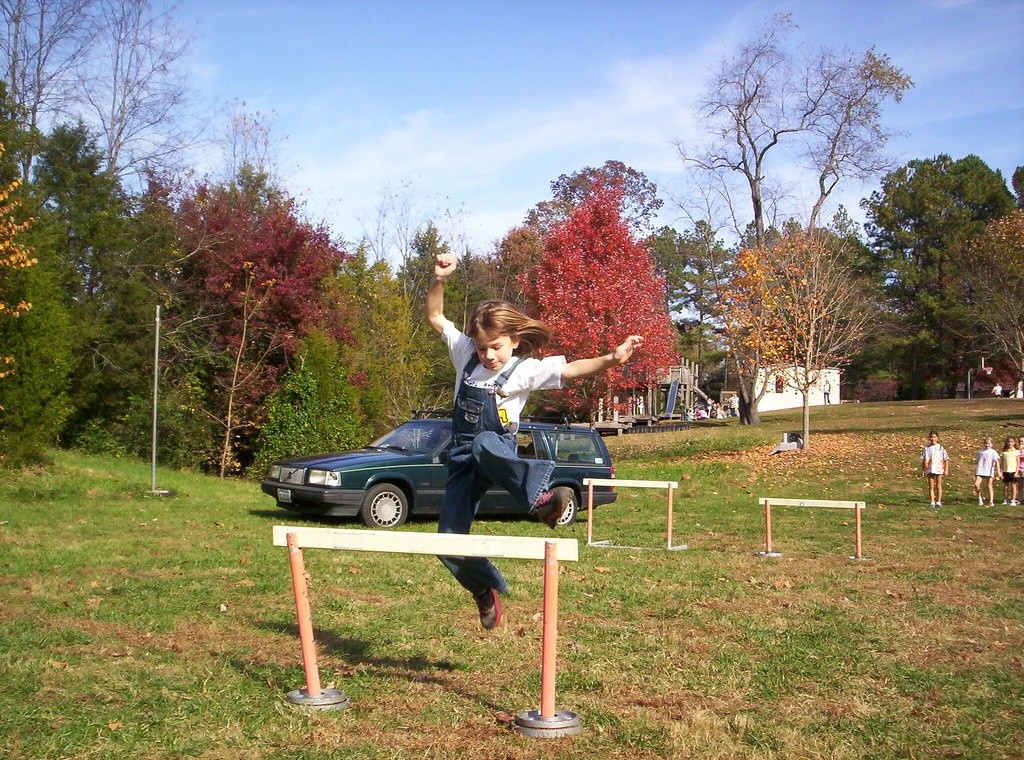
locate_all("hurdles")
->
[757,497,867,560]
[581,479,689,552]
[269,524,584,739]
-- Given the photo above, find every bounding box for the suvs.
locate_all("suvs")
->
[261,408,620,529]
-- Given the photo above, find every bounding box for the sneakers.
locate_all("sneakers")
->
[478,589,501,630]
[532,487,573,529]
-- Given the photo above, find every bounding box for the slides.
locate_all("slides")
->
[664,381,679,419]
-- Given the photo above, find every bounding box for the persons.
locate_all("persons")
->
[424,252,645,629]
[678,393,740,421]
[921,431,949,507]
[824,380,831,405]
[999,435,1024,507]
[638,396,643,415]
[974,437,1001,507]
[991,383,1002,398]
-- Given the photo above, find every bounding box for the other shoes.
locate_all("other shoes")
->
[931,502,941,508]
[1002,498,1016,506]
[978,497,983,506]
[986,503,994,508]
